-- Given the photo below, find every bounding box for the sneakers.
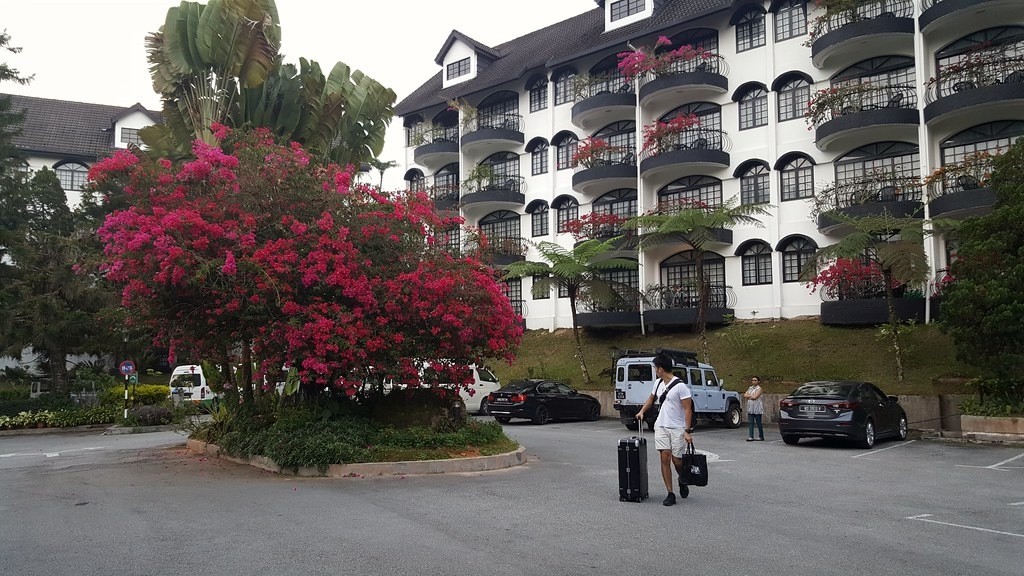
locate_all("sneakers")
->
[678,477,689,498]
[663,492,676,506]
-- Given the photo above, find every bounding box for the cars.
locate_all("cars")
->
[488,379,602,427]
[778,380,909,451]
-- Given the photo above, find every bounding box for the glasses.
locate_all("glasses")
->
[752,380,757,381]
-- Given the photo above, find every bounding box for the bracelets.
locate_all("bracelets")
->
[685,428,692,433]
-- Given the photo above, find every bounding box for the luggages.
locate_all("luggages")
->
[617,416,649,503]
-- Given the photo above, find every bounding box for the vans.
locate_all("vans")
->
[169,364,244,415]
[253,358,501,416]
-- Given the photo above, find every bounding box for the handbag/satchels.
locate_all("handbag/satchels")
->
[649,407,661,432]
[681,440,709,486]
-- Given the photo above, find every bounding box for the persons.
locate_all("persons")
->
[634,355,694,507]
[744,376,765,441]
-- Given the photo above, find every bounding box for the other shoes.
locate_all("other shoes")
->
[754,437,764,441]
[747,437,754,441]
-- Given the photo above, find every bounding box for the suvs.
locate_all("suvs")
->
[613,348,744,433]
[28,377,101,407]
